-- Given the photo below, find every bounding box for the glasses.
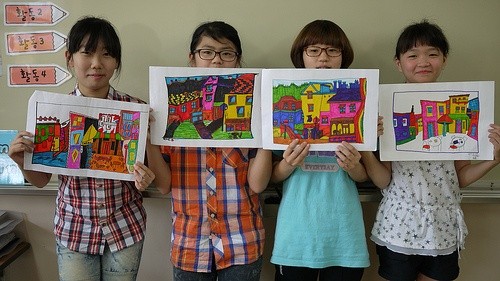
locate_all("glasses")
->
[193,49,238,62]
[303,45,343,57]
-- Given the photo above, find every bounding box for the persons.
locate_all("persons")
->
[270,19,373,281]
[8,17,157,281]
[145,20,274,281]
[358,21,500,281]
